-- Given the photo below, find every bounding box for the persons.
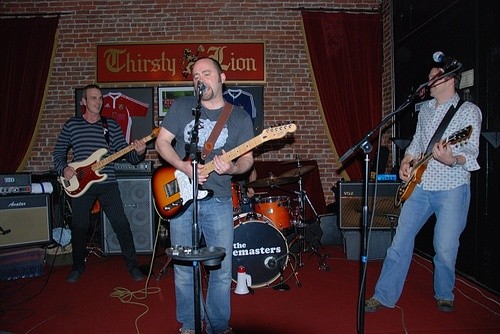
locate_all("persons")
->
[53,85,146,283]
[155,57,254,334]
[362,57,482,313]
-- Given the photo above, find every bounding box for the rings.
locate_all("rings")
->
[217,167,220,170]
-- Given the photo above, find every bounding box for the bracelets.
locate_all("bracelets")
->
[448,156,458,167]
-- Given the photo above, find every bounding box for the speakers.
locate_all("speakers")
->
[0,193,53,250]
[101,176,156,255]
[335,180,404,230]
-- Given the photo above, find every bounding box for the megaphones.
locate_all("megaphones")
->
[234,265,252,294]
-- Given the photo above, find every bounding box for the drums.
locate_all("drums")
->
[231,212,289,288]
[252,197,295,236]
[230,182,242,212]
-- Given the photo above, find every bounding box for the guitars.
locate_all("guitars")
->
[398,126,473,200]
[151,121,298,220]
[59,127,161,198]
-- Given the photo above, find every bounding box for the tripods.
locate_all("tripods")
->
[288,163,330,273]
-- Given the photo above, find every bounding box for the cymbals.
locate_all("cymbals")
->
[282,166,317,177]
[280,157,311,165]
[242,177,294,187]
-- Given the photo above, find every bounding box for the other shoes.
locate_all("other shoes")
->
[364,298,385,313]
[438,299,455,313]
[129,268,145,280]
[68,267,84,283]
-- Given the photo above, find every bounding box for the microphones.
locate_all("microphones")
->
[3,229,11,235]
[433,51,462,67]
[198,81,208,92]
[268,259,276,267]
